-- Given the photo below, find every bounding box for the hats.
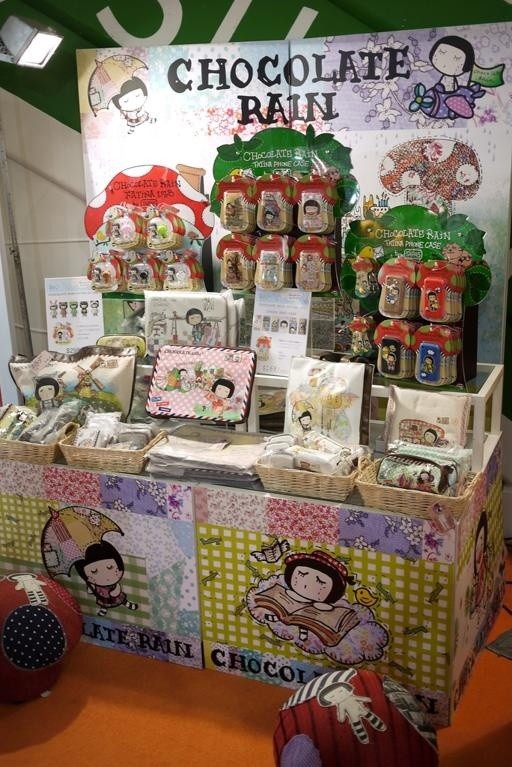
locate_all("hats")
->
[283,549,348,591]
